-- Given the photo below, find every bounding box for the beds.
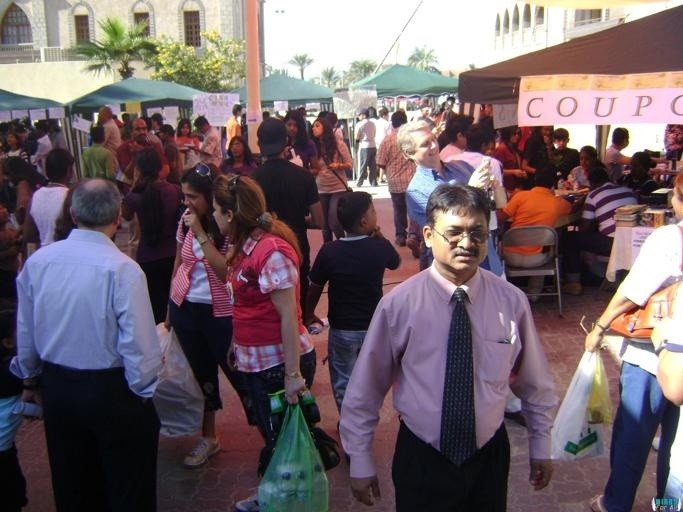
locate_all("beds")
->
[624,167,681,182]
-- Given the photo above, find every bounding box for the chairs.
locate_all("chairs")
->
[582,179,661,296]
[639,181,660,197]
[502,225,562,318]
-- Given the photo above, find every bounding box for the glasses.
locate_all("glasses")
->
[229,174,240,196]
[429,225,489,245]
[193,161,214,184]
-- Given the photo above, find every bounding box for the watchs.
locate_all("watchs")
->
[594,316,609,333]
[281,369,304,382]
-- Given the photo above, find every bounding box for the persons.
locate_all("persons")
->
[216,136,257,180]
[222,103,244,158]
[212,172,317,512]
[149,111,163,139]
[300,191,401,416]
[547,128,579,191]
[493,125,536,191]
[45,118,68,151]
[120,148,187,324]
[446,122,507,280]
[497,165,587,303]
[96,106,119,156]
[395,118,509,284]
[558,167,634,296]
[18,148,75,275]
[250,118,325,312]
[115,117,171,199]
[405,114,474,272]
[9,179,166,512]
[0,132,28,165]
[585,168,683,511]
[158,124,185,185]
[161,161,258,469]
[353,104,393,190]
[337,181,561,512]
[521,126,552,187]
[30,122,52,184]
[603,128,631,185]
[284,109,321,179]
[3,155,49,225]
[650,280,683,512]
[0,344,43,512]
[183,116,224,170]
[79,125,120,183]
[557,145,612,192]
[374,111,417,246]
[175,117,200,170]
[613,152,660,197]
[310,117,354,245]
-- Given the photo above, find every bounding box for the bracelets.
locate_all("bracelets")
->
[197,236,208,246]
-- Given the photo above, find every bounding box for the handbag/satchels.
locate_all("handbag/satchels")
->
[608,226,683,339]
[319,149,352,193]
[175,183,188,234]
[256,394,341,481]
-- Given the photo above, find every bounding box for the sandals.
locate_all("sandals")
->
[589,495,606,511]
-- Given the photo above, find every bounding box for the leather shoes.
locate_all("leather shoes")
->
[503,410,526,428]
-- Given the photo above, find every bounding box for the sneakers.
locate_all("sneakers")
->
[563,281,582,295]
[527,291,540,301]
[406,238,420,258]
[396,234,405,245]
[183,436,221,467]
[235,495,260,511]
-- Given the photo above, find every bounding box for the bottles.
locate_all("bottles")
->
[312,463,329,512]
[277,473,296,512]
[297,470,312,512]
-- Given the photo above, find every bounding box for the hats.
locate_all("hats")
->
[358,108,368,115]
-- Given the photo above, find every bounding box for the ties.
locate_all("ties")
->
[440,288,478,468]
